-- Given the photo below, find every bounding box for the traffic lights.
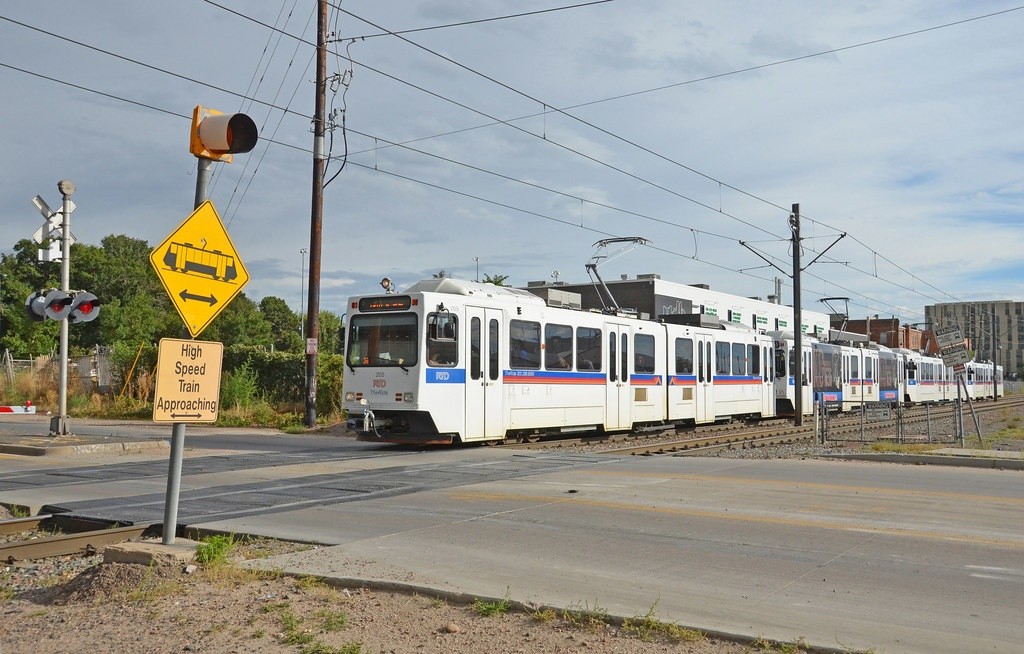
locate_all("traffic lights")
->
[77,298,100,314]
[188,100,259,164]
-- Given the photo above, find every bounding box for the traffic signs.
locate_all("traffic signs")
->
[49,295,75,312]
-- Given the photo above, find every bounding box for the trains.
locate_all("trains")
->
[339,271,1008,442]
